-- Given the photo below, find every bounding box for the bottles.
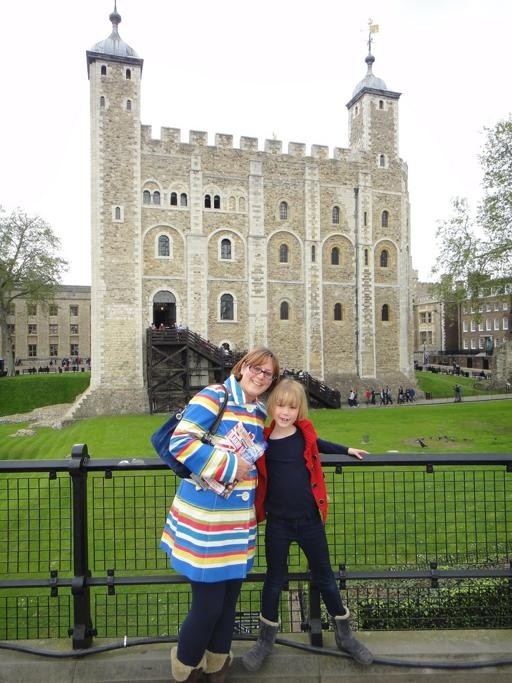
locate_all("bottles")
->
[241,441,267,463]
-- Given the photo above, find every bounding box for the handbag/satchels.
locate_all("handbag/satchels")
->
[150,383,229,479]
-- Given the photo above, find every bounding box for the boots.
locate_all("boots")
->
[169,645,235,683]
[326,605,375,666]
[242,612,282,672]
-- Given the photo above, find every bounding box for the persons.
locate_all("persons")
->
[242,379,374,672]
[150,347,280,683]
[452,385,464,402]
[348,385,415,408]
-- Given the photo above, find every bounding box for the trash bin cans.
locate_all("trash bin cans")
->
[426,393,430,399]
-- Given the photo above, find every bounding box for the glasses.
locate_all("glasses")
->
[247,364,277,380]
